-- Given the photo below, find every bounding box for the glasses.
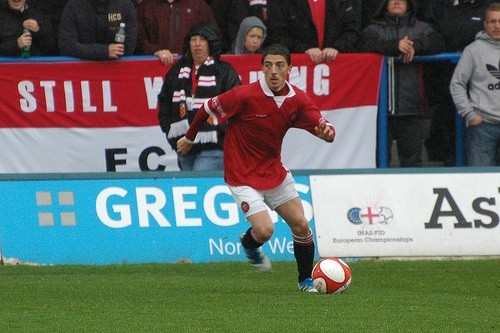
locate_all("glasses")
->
[484,19,499,25]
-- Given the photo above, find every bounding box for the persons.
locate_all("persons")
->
[0,0,58,57]
[157,25,241,172]
[204,0,250,54]
[267,0,363,64]
[449,2,500,168]
[138,0,223,66]
[176,44,336,293]
[360,2,441,167]
[225,16,267,54]
[58,0,138,60]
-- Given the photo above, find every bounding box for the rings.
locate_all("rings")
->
[162,54,166,57]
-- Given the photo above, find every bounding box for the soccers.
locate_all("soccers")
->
[312,258,352,294]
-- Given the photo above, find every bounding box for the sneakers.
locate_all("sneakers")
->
[240,232,271,274]
[297,277,319,293]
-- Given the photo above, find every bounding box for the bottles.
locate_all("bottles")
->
[20,28,31,58]
[114,22,126,44]
